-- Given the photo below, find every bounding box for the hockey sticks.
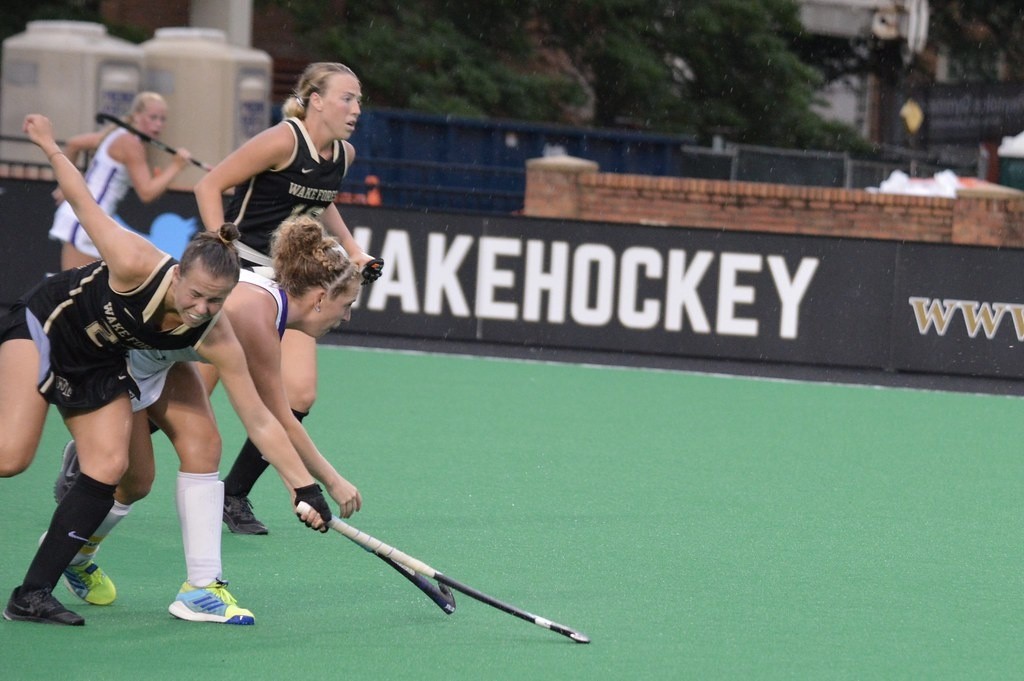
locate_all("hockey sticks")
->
[95,112,213,172]
[294,500,592,645]
[345,538,457,616]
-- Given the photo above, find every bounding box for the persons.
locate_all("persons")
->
[54,62,384,534]
[39,215,360,608]
[50,92,193,271]
[0,113,332,626]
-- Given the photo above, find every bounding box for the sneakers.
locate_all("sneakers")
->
[53,439,80,505]
[222,495,268,535]
[39,531,116,605]
[168,580,255,625]
[3,586,84,626]
